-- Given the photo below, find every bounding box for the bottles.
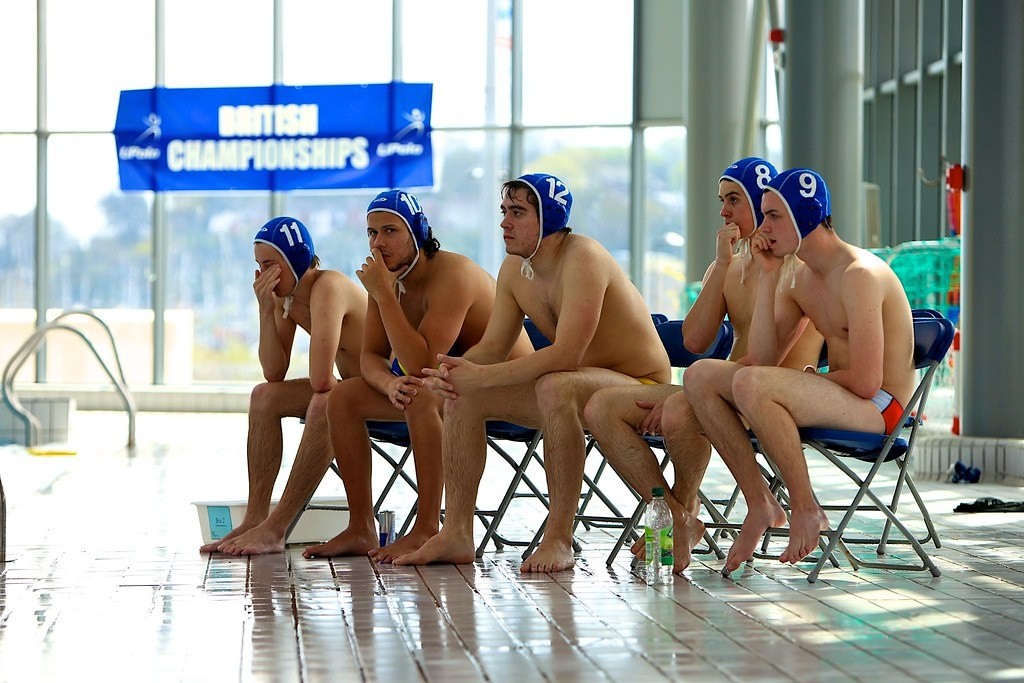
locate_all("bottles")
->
[644,487,674,586]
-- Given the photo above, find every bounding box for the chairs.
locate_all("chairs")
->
[300,309,955,583]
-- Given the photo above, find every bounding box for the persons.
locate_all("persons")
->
[683,168,915,578]
[584,157,826,573]
[199,216,408,557]
[302,190,536,563]
[392,173,672,572]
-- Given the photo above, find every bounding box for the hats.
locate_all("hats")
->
[718,157,779,284]
[764,168,832,292]
[514,173,573,280]
[366,190,428,304]
[253,216,315,319]
